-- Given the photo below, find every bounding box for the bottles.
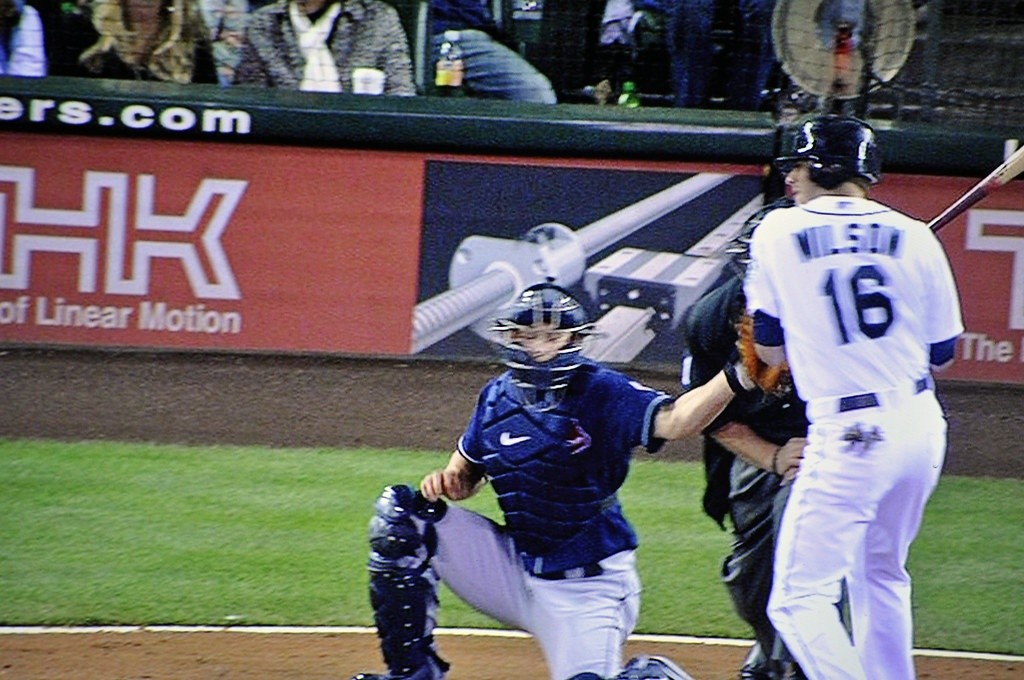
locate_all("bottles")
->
[618,80,641,109]
[436,30,463,97]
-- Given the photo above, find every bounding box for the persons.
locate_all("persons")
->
[1,1,637,104]
[352,278,754,680]
[682,113,965,679]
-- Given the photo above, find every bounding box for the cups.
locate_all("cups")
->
[353,68,386,95]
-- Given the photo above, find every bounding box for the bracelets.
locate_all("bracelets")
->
[724,360,746,394]
[772,444,781,477]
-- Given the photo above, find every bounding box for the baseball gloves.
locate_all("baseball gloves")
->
[735,311,777,392]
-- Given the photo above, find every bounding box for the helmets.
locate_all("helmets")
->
[776,113,882,185]
[491,283,586,392]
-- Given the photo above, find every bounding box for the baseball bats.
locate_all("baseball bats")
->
[934,143,1024,227]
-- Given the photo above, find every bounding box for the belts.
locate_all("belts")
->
[534,562,605,580]
[838,378,927,413]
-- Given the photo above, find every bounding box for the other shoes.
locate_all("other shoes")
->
[638,655,691,680]
